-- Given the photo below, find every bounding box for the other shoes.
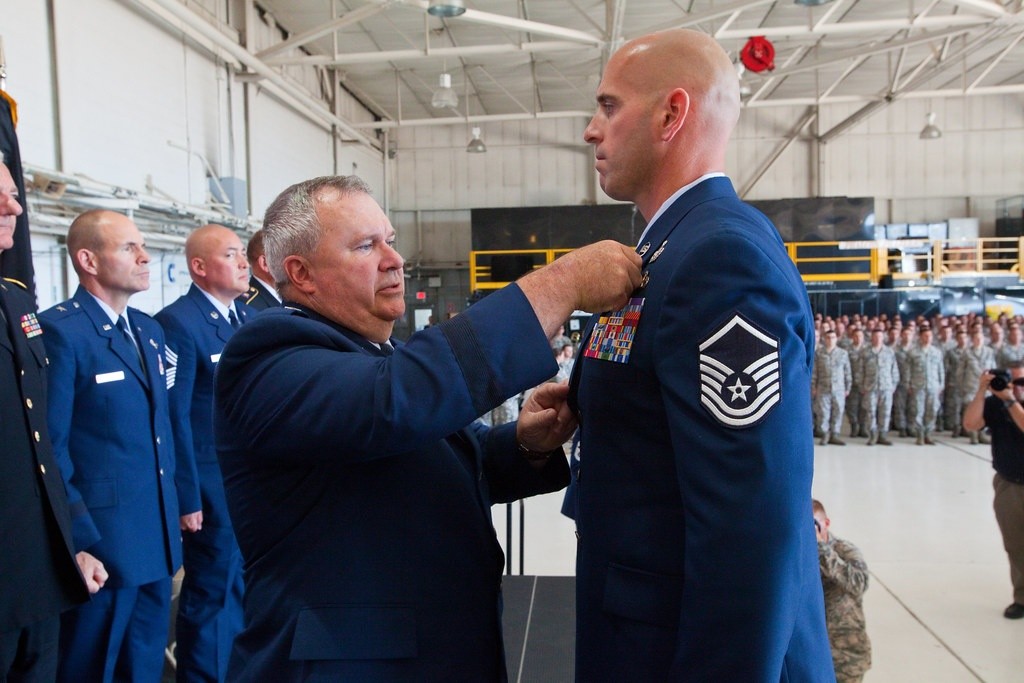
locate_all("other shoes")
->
[1004,602,1024,621]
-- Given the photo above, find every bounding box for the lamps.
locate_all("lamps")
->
[466,65,486,153]
[919,86,940,137]
[426,0,466,17]
[432,25,459,107]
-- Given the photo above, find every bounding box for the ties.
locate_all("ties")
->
[228,310,241,331]
[115,315,146,373]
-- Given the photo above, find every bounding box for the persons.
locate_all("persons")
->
[214,175,642,683]
[234,229,282,314]
[153,226,266,683]
[36,209,184,683]
[963,360,1024,618]
[0,89,109,683]
[811,311,1024,446]
[423,313,579,428]
[561,28,839,683]
[813,499,872,683]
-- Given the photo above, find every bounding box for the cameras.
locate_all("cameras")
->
[989,368,1012,391]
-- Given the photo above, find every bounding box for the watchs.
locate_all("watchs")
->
[1003,399,1017,409]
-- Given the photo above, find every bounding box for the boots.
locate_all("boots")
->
[899,429,918,437]
[820,434,845,446]
[917,432,935,445]
[970,432,992,444]
[850,424,869,437]
[867,432,893,445]
[952,426,971,438]
